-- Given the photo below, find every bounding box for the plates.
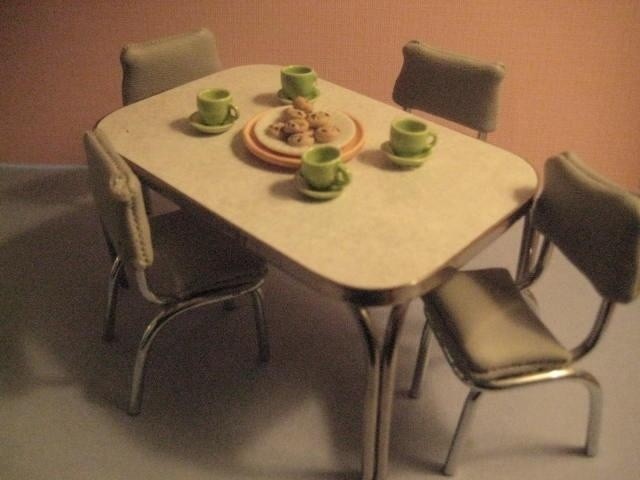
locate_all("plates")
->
[188,111,234,134]
[276,88,321,102]
[240,99,368,170]
[295,170,343,199]
[380,140,432,167]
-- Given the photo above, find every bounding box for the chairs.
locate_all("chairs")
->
[80,129,271,416]
[410,151,640,476]
[392,43,506,145]
[118,29,222,108]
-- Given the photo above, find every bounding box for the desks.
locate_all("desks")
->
[92,57,541,480]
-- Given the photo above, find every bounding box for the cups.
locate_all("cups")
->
[389,117,438,158]
[197,89,240,124]
[281,66,322,100]
[302,144,351,191]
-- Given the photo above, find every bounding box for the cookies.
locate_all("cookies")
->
[268,96,340,146]
